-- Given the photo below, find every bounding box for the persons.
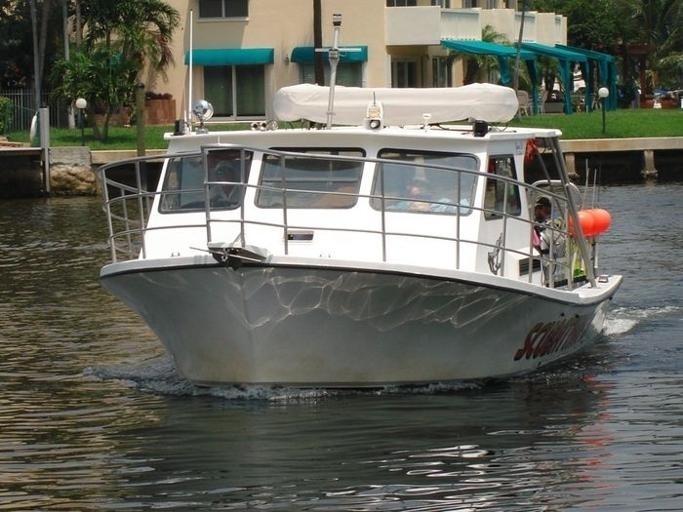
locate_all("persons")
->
[204,159,246,204]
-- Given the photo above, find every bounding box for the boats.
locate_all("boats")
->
[91,11,624,400]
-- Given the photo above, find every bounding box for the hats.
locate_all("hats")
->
[529,194,553,208]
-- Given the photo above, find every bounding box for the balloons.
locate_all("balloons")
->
[567,208,611,236]
